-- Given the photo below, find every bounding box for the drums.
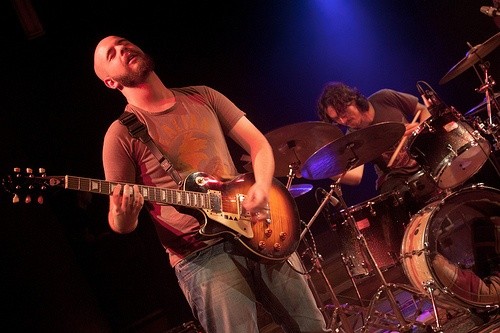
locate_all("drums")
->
[399,183,500,313]
[326,186,419,277]
[405,169,447,205]
[404,106,491,190]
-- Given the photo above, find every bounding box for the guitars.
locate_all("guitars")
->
[1,168,301,263]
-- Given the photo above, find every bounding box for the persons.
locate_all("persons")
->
[94,36,332,333]
[317,83,433,256]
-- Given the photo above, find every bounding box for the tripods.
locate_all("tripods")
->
[299,182,483,333]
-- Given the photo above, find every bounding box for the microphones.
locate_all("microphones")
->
[318,188,340,207]
[424,120,438,135]
[416,83,436,114]
[480,5,500,17]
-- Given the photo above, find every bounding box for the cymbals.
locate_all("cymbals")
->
[264,121,344,178]
[464,92,500,114]
[438,31,500,84]
[302,121,405,180]
[287,184,313,198]
